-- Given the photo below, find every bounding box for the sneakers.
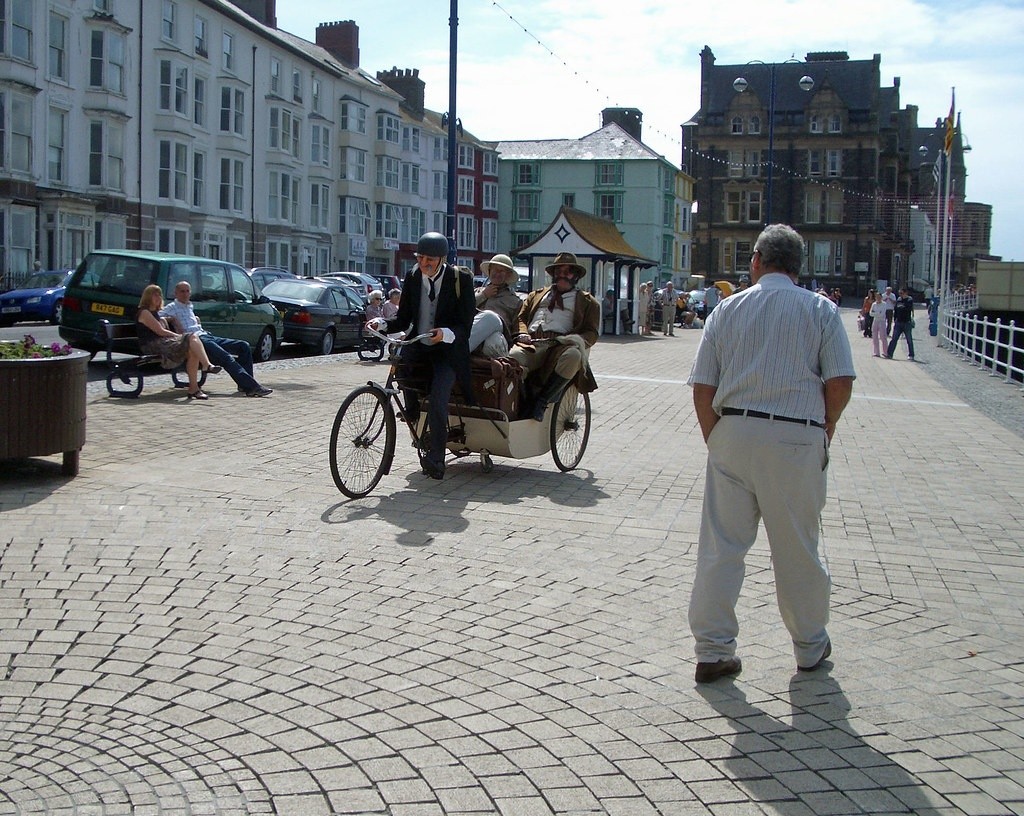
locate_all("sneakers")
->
[237,385,273,397]
[797,639,831,672]
[696,655,742,683]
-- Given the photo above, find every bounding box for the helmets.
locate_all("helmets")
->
[417,232,449,257]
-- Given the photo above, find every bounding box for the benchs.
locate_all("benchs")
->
[349,310,387,360]
[93,319,208,397]
[603,316,615,333]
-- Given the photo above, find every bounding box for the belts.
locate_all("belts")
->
[721,407,826,430]
[663,303,675,306]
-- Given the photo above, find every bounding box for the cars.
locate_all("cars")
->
[689,290,708,318]
[59,246,283,368]
[260,277,374,357]
[0,268,101,323]
[245,260,403,300]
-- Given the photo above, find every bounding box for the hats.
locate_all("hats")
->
[739,275,749,282]
[545,253,586,279]
[480,254,519,284]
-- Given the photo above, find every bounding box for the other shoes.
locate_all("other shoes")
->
[396,409,420,421]
[872,354,880,358]
[882,353,892,359]
[624,331,632,336]
[624,321,635,325]
[432,462,445,479]
[907,356,914,360]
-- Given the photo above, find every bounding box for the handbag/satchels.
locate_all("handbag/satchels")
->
[858,313,867,331]
[910,318,915,328]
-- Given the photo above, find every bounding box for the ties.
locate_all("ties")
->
[548,285,575,313]
[428,265,444,302]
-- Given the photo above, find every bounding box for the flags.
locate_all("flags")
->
[931,91,956,219]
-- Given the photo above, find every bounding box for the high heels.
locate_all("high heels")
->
[187,391,209,399]
[201,364,222,376]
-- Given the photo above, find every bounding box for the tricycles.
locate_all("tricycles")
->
[328,305,595,498]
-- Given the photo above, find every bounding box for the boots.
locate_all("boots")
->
[518,369,571,422]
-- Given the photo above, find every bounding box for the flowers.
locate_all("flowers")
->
[0,333,72,359]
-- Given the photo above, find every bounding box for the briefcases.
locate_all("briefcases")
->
[449,356,519,422]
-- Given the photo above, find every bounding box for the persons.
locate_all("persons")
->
[467,254,524,359]
[686,225,856,684]
[602,290,635,335]
[795,277,842,306]
[509,252,601,422]
[157,281,273,397]
[924,285,975,309]
[136,284,222,399]
[640,275,749,336]
[366,289,413,342]
[861,287,916,361]
[27,261,46,283]
[365,232,476,480]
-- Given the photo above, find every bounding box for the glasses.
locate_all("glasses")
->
[553,269,571,276]
[747,250,762,262]
[375,297,383,301]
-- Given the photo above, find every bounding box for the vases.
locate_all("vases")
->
[1,340,91,476]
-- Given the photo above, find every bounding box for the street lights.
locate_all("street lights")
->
[919,131,973,285]
[734,59,816,230]
